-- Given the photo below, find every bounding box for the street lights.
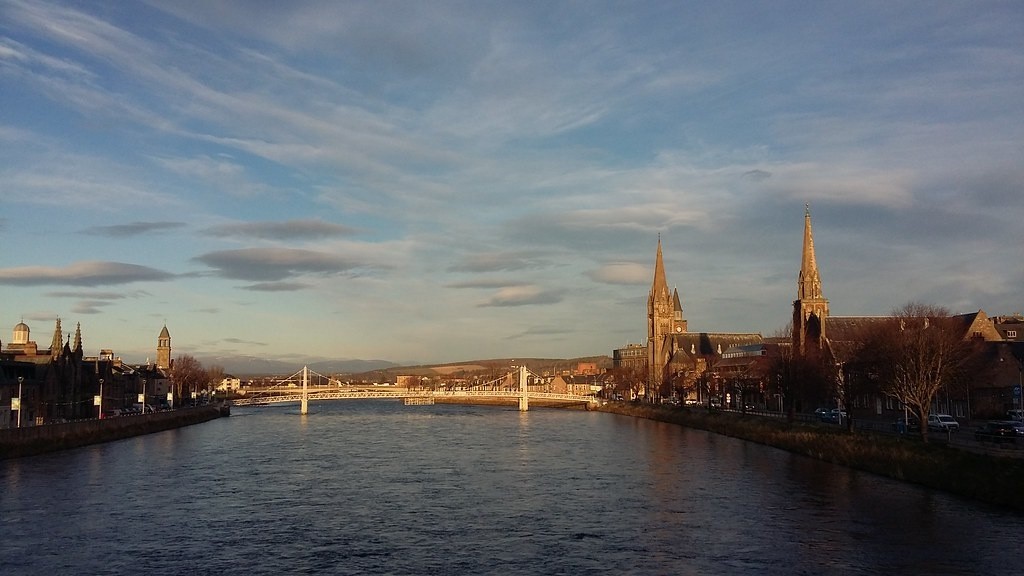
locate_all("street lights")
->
[99,378,105,420]
[17,376,24,428]
[142,379,146,415]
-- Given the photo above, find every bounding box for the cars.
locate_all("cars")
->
[685,399,698,405]
[831,407,848,419]
[890,416,918,434]
[974,420,1024,444]
[814,408,832,417]
[927,414,959,434]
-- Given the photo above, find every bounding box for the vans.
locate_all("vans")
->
[711,398,721,409]
